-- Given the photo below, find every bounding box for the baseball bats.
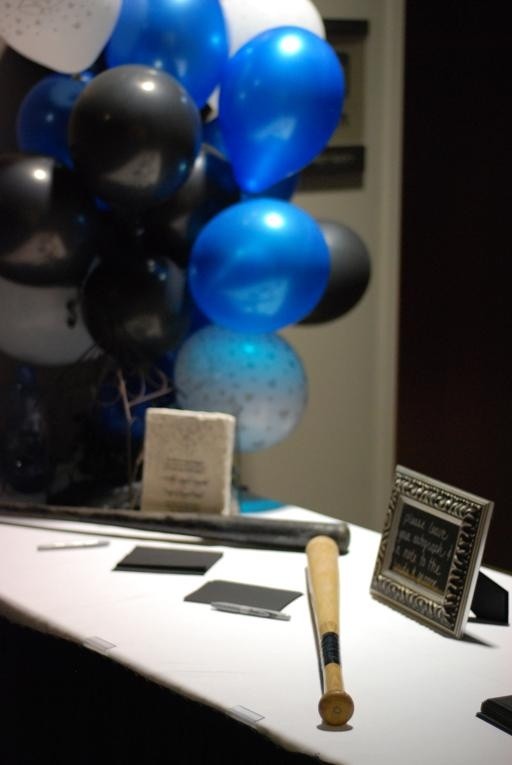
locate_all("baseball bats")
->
[305,535,354,725]
[0,501,349,555]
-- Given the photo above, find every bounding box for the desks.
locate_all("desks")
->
[1,502,512,764]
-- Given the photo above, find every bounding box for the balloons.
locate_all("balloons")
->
[2,1,371,455]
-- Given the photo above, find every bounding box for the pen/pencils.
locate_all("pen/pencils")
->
[37,540,108,551]
[211,600,290,620]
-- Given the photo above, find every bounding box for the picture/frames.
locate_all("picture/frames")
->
[369,464,495,638]
